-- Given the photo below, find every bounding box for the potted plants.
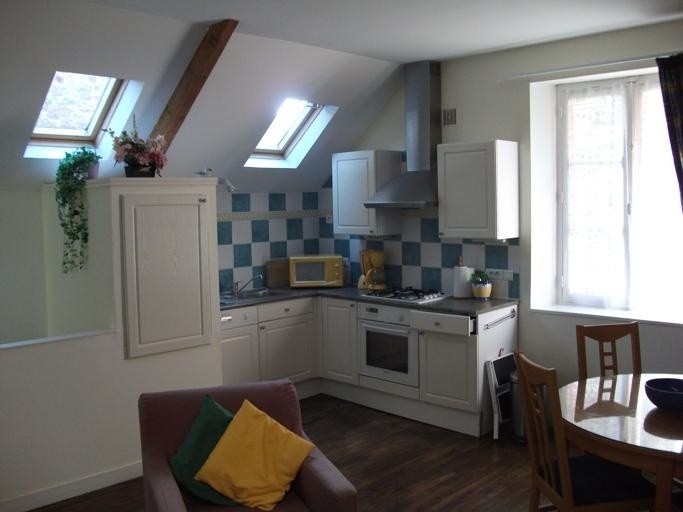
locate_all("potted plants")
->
[56,149,102,275]
[472,270,492,298]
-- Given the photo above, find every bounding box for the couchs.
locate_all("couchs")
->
[138,377,358,512]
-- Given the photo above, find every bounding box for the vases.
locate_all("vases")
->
[126,166,154,178]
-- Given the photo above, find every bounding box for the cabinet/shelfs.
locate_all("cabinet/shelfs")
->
[332,150,402,236]
[42,175,220,360]
[437,139,521,240]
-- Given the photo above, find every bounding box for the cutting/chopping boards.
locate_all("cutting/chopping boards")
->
[266,259,290,288]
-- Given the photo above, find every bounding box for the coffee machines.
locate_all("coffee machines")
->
[357,250,388,291]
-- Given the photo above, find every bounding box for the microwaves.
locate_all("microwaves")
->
[289,256,344,289]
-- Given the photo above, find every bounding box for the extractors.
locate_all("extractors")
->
[363,62,439,210]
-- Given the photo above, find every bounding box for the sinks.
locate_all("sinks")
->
[233,290,273,298]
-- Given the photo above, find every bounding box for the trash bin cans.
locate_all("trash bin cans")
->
[510,369,551,438]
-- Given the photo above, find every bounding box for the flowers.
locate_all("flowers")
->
[102,113,169,178]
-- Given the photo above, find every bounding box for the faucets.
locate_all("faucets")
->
[232,274,263,294]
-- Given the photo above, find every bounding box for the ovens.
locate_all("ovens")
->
[356,319,419,389]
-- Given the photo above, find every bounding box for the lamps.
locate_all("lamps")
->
[218,176,236,194]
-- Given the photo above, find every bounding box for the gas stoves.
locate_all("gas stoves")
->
[362,286,451,305]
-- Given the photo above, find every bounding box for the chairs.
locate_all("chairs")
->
[515,352,655,511]
[575,320,642,378]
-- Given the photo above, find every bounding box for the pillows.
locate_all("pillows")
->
[193,398,316,512]
[168,393,234,507]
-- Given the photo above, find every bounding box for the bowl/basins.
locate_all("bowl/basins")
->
[644,378,682,410]
